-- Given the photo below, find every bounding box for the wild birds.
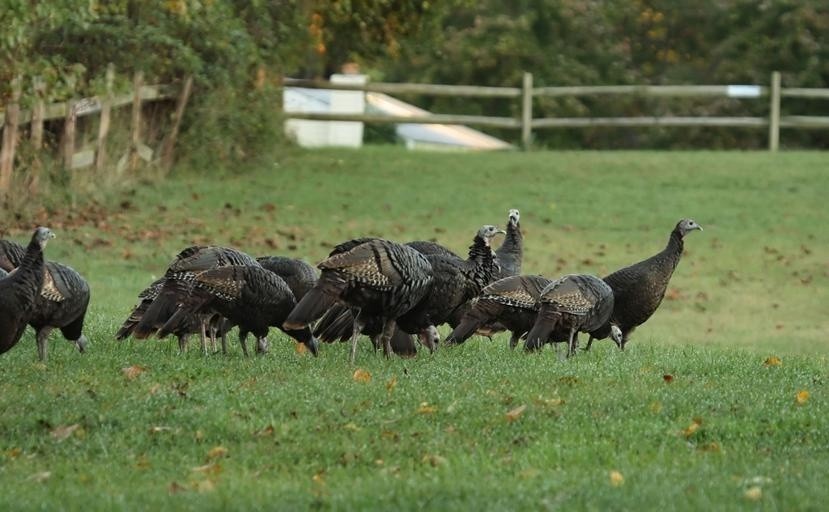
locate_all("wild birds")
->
[114,207,623,368]
[0,226,57,355]
[583,216,704,352]
[0,240,90,361]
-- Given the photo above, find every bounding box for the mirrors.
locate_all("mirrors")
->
[0,240,90,361]
[0,226,57,355]
[583,216,704,352]
